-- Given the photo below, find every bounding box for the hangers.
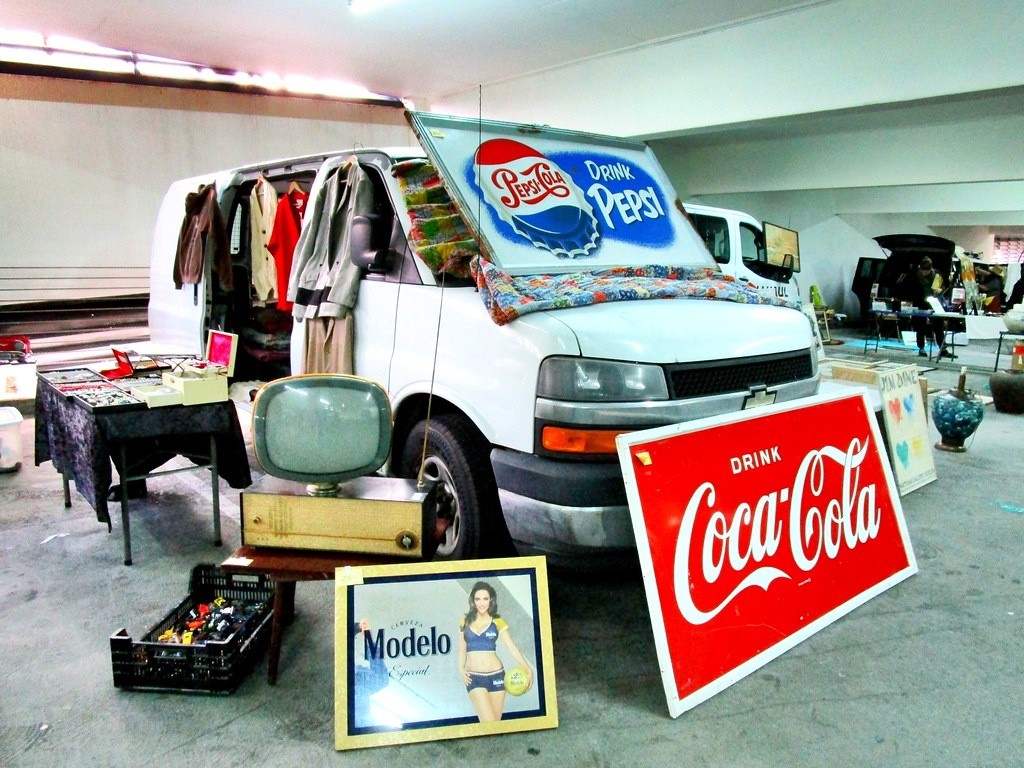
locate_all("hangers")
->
[338,141,364,183]
[256,169,265,190]
[288,168,305,197]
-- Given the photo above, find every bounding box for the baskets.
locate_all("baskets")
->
[109,563,296,696]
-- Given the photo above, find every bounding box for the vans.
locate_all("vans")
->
[681,200,803,313]
[851,232,991,337]
[145,146,823,587]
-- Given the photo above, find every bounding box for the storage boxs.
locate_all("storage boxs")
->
[1012,338,1024,371]
[0,406,24,473]
[36,347,171,414]
[110,564,296,695]
[130,386,183,409]
[161,329,238,406]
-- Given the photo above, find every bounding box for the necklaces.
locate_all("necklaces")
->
[50,375,162,395]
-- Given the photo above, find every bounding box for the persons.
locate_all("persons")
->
[976,264,1024,313]
[901,255,958,359]
[459,581,533,722]
[353,619,391,731]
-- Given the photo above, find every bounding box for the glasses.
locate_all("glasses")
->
[977,268,982,272]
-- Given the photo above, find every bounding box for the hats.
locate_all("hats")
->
[920,256,933,270]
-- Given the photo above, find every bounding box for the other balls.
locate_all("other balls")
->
[504,668,530,696]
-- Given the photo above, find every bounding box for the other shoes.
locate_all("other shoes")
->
[941,350,958,358]
[919,349,927,357]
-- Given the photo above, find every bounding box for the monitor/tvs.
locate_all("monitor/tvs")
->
[252,373,394,495]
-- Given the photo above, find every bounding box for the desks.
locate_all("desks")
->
[222,518,454,687]
[863,309,965,364]
[993,330,1024,372]
[814,306,837,341]
[35,378,254,563]
